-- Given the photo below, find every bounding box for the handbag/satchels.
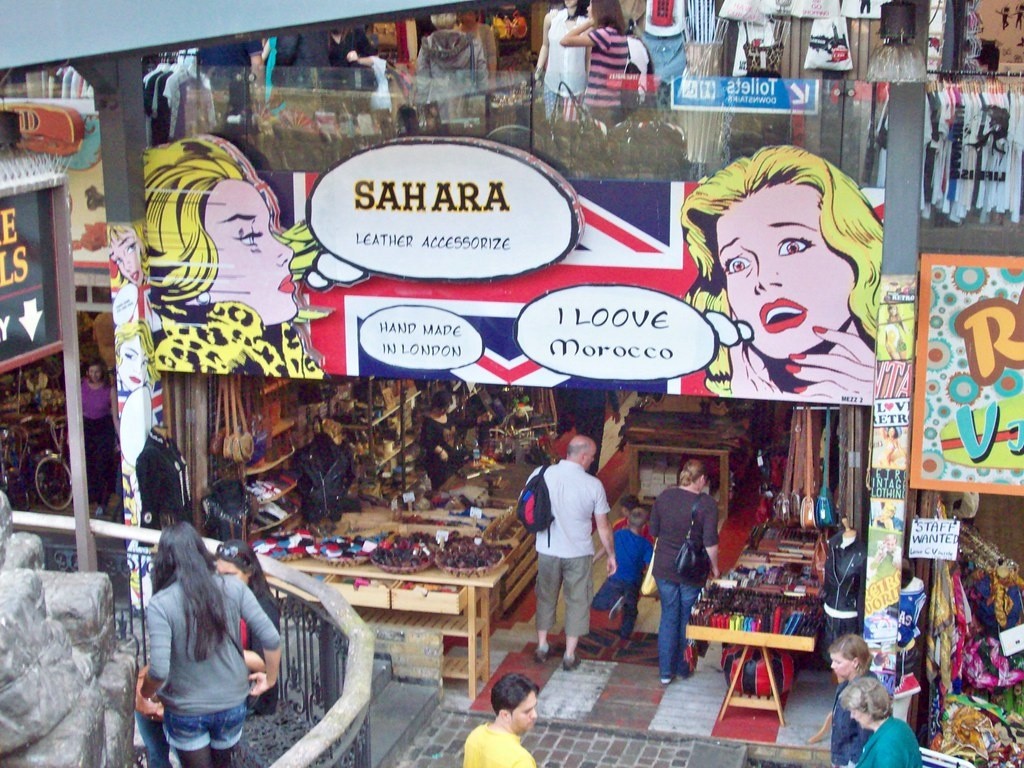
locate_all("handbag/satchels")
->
[211,428,254,461]
[773,491,834,529]
[671,492,710,582]
[641,537,659,595]
[717,0,891,77]
[349,64,378,91]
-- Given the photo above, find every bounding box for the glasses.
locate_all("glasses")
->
[217,544,250,565]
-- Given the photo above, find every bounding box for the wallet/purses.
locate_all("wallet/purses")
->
[686,518,826,637]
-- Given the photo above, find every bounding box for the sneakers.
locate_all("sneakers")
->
[893,673,921,699]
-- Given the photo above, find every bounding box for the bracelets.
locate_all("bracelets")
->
[438,448,444,454]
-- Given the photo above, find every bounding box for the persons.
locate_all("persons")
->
[416,10,497,131]
[840,678,922,768]
[649,460,720,683]
[830,634,877,768]
[136,423,193,529]
[81,359,119,516]
[420,392,456,489]
[196,23,375,116]
[137,522,280,768]
[879,426,907,467]
[526,436,617,669]
[463,672,540,768]
[593,496,654,640]
[966,38,1000,72]
[560,0,629,129]
[824,520,865,645]
[533,0,593,130]
[566,389,622,475]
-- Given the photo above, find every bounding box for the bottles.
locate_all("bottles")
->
[473,439,480,465]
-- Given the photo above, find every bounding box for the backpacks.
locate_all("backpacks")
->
[301,414,353,522]
[516,464,555,532]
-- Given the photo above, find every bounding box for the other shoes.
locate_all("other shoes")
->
[533,642,559,663]
[608,594,625,619]
[347,382,416,499]
[661,675,671,684]
[563,653,582,670]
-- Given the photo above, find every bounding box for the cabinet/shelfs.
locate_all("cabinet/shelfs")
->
[625,440,731,533]
[282,554,510,701]
[245,377,303,542]
[326,376,423,506]
